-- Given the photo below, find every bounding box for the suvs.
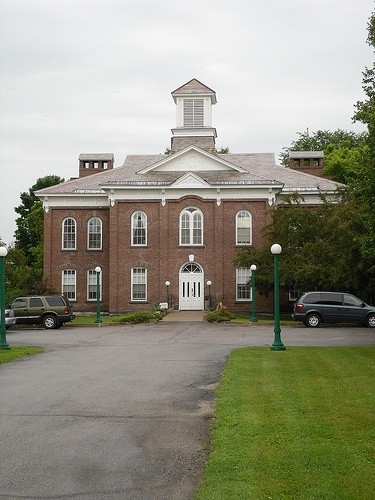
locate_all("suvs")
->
[292,291,375,329]
[6,294,78,328]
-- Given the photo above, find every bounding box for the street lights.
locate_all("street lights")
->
[0,247,12,349]
[207,280,214,311]
[94,266,103,323]
[165,280,171,308]
[250,264,257,322]
[270,244,287,351]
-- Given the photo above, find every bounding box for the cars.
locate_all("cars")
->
[3,309,17,329]
